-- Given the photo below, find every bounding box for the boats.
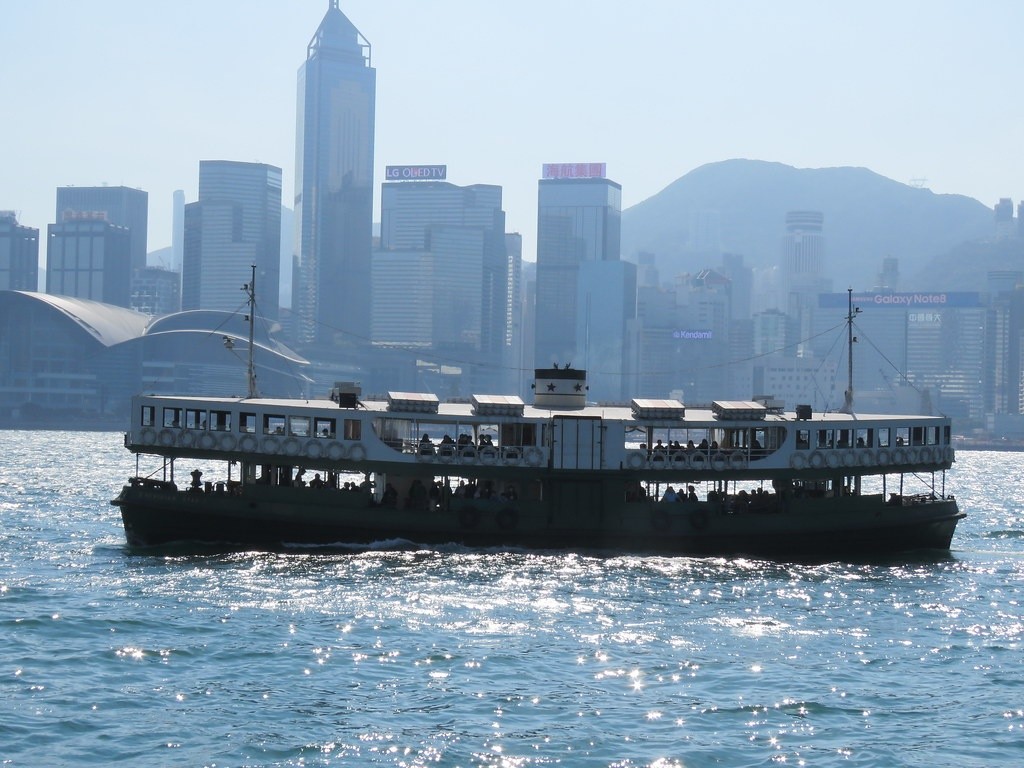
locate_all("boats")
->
[107,264,966,561]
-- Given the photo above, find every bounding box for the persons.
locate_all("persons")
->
[731,440,762,461]
[827,440,841,448]
[201,420,206,430]
[382,477,519,512]
[420,434,434,454]
[172,419,179,428]
[458,434,475,457]
[896,437,903,447]
[322,428,331,439]
[858,437,872,448]
[341,475,375,495]
[792,481,803,497]
[441,435,456,455]
[797,439,808,450]
[717,488,775,514]
[653,439,723,462]
[227,422,231,432]
[273,427,293,435]
[478,434,493,456]
[295,467,325,489]
[640,486,698,504]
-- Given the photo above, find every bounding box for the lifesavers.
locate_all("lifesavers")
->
[808,450,826,468]
[860,448,876,466]
[906,448,920,465]
[842,450,860,467]
[139,427,368,463]
[892,448,906,467]
[730,451,747,473]
[652,510,672,531]
[711,451,729,472]
[496,507,519,531]
[689,509,708,530]
[627,451,707,471]
[944,445,954,462]
[920,446,933,465]
[417,444,546,468]
[458,505,481,530]
[826,450,842,467]
[876,448,892,467]
[933,447,944,465]
[790,452,808,471]
